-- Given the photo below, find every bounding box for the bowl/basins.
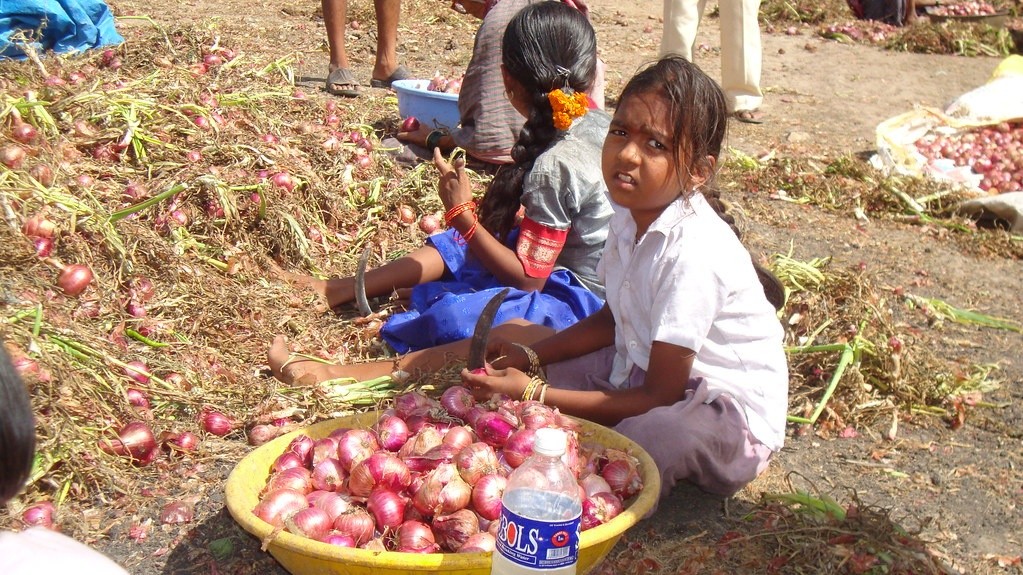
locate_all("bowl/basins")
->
[927,9,1009,30]
[392,79,461,130]
[223,409,660,575]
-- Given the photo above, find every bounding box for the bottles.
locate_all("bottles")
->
[491,427,582,575]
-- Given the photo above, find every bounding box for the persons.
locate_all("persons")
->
[657,0,768,124]
[267,0,617,354]
[382,0,603,175]
[0,340,132,575]
[320,0,419,100]
[267,56,790,522]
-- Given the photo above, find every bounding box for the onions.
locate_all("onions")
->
[931,0,997,17]
[252,367,642,552]
[917,121,1023,195]
[766,2,898,42]
[1,46,465,531]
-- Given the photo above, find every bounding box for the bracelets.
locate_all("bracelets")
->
[425,129,447,151]
[518,374,549,406]
[512,342,543,377]
[444,203,478,246]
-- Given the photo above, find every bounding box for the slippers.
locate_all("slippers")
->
[370,66,411,88]
[326,68,366,96]
[734,109,765,125]
